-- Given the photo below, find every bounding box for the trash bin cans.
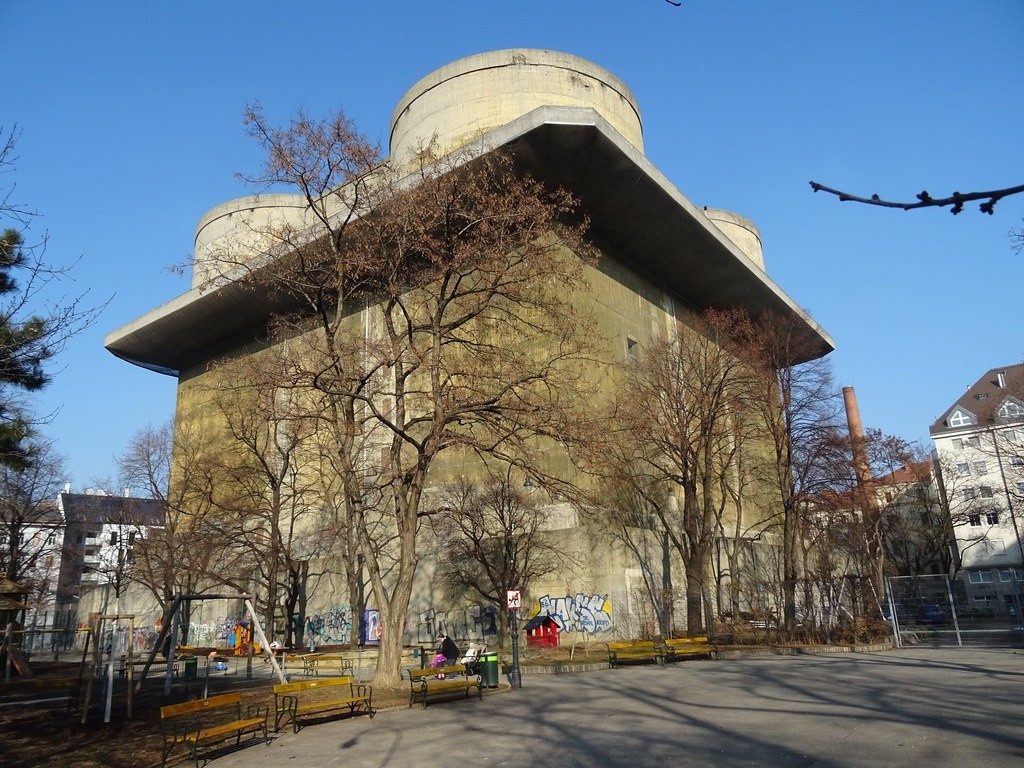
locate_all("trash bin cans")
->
[184,658,198,680]
[310,640,315,652]
[479,651,498,687]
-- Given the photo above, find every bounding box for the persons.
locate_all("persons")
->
[433,648,448,680]
[438,634,460,679]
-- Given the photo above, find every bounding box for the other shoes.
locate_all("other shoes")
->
[445,676,453,680]
[452,675,457,678]
[436,677,444,680]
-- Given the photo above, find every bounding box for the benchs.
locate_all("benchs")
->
[160,691,269,768]
[665,637,717,665]
[605,641,664,669]
[271,656,354,679]
[273,675,373,734]
[406,665,483,711]
